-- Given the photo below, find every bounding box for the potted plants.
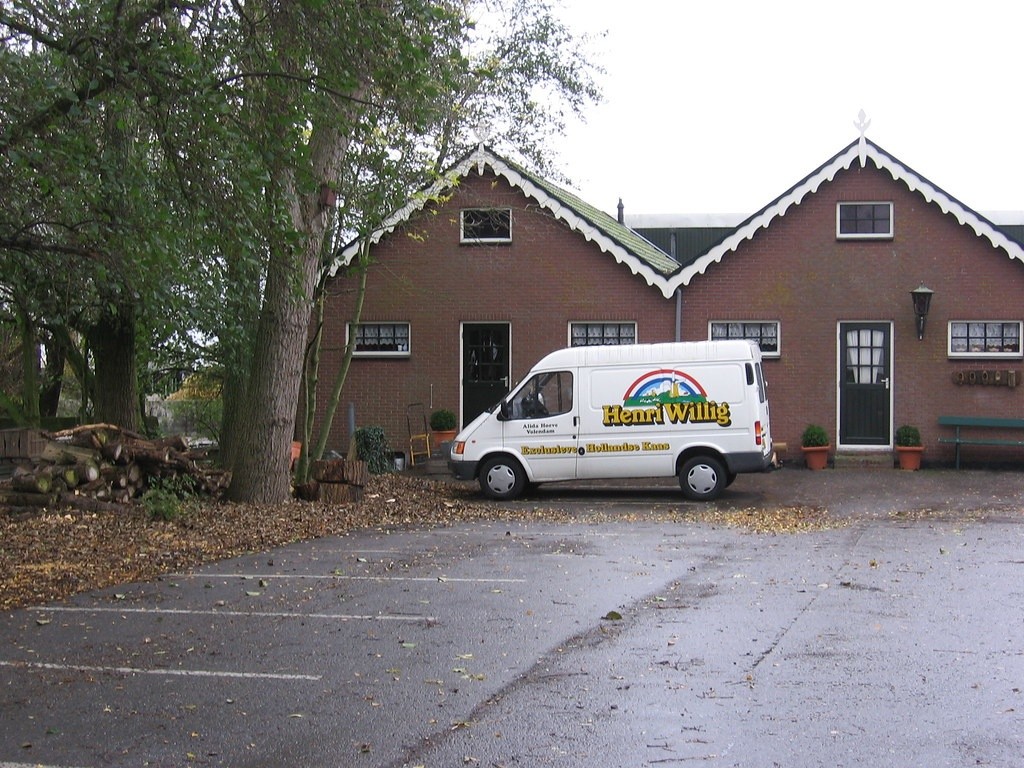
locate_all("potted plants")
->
[895,424,924,471]
[800,425,832,469]
[429,409,457,448]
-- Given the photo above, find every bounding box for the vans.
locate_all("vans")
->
[445,335,784,503]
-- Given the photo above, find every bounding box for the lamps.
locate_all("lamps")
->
[909,280,935,342]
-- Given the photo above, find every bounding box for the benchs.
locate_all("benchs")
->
[938,416,1024,470]
